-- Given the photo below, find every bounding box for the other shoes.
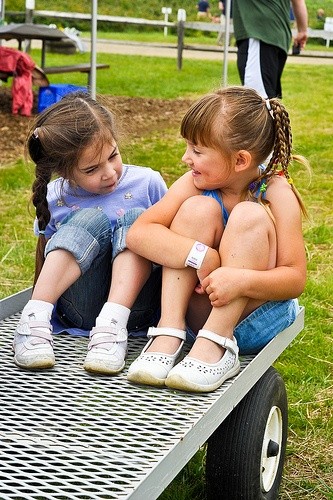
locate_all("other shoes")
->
[12,320,55,368]
[83,326,129,375]
[127,326,240,392]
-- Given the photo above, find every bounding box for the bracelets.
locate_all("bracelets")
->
[184,241,209,270]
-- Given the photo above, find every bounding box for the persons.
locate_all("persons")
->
[216,0,232,45]
[208,13,220,23]
[126,86,308,393]
[290,1,296,27]
[12,90,168,375]
[316,8,325,20]
[232,0,308,164]
[195,0,210,20]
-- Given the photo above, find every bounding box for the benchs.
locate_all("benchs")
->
[0,63,110,92]
